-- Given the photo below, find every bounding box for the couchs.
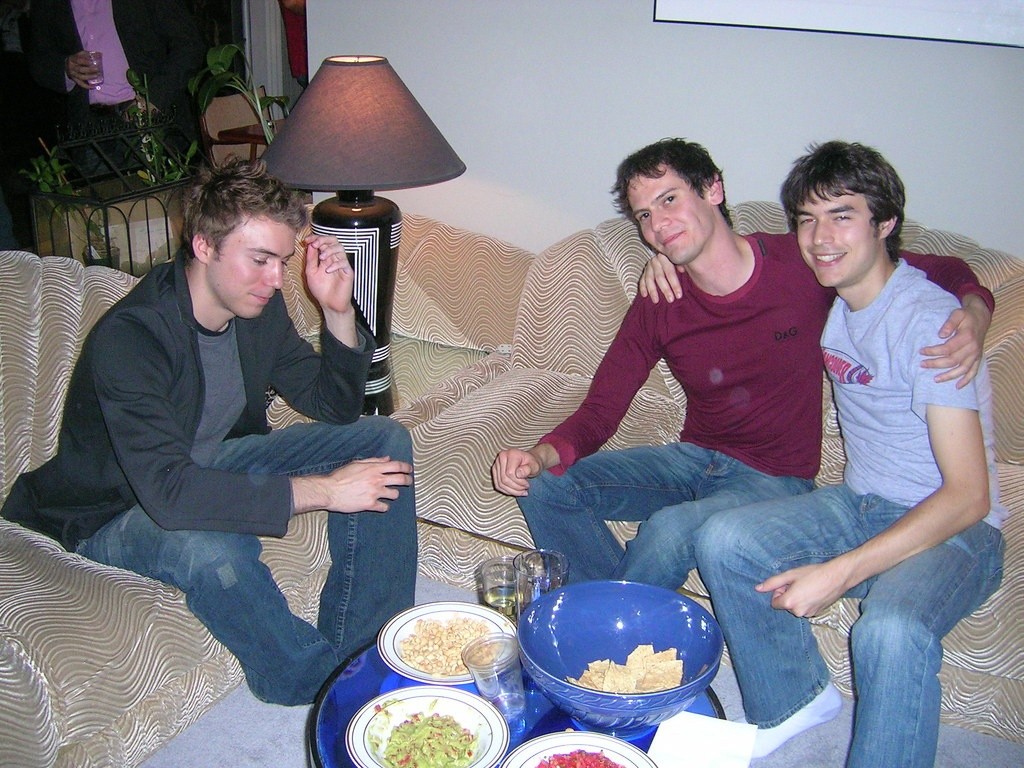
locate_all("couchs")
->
[0,248,334,768]
[224,199,1024,743]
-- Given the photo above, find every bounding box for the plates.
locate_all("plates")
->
[344,685,510,768]
[377,600,518,686]
[498,730,658,768]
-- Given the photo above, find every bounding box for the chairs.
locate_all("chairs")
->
[194,85,271,171]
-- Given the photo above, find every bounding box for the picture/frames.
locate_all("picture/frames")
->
[653,0,1024,49]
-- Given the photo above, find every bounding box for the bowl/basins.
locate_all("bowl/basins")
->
[515,580,724,741]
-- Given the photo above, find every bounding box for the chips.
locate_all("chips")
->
[565,644,683,694]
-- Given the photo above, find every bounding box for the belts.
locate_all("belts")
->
[88,99,134,118]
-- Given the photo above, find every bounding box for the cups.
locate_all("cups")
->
[512,550,571,626]
[461,631,527,738]
[481,556,529,628]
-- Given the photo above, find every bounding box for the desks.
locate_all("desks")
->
[218,118,286,162]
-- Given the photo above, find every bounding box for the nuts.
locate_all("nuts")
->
[399,617,503,677]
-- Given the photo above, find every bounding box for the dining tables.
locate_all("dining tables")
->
[305,641,724,768]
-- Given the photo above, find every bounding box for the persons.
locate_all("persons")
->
[0,158,419,709]
[491,136,995,592]
[17,0,205,158]
[640,143,1007,768]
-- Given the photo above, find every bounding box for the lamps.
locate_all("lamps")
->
[261,54,468,417]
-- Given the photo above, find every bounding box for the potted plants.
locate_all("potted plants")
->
[18,136,120,269]
